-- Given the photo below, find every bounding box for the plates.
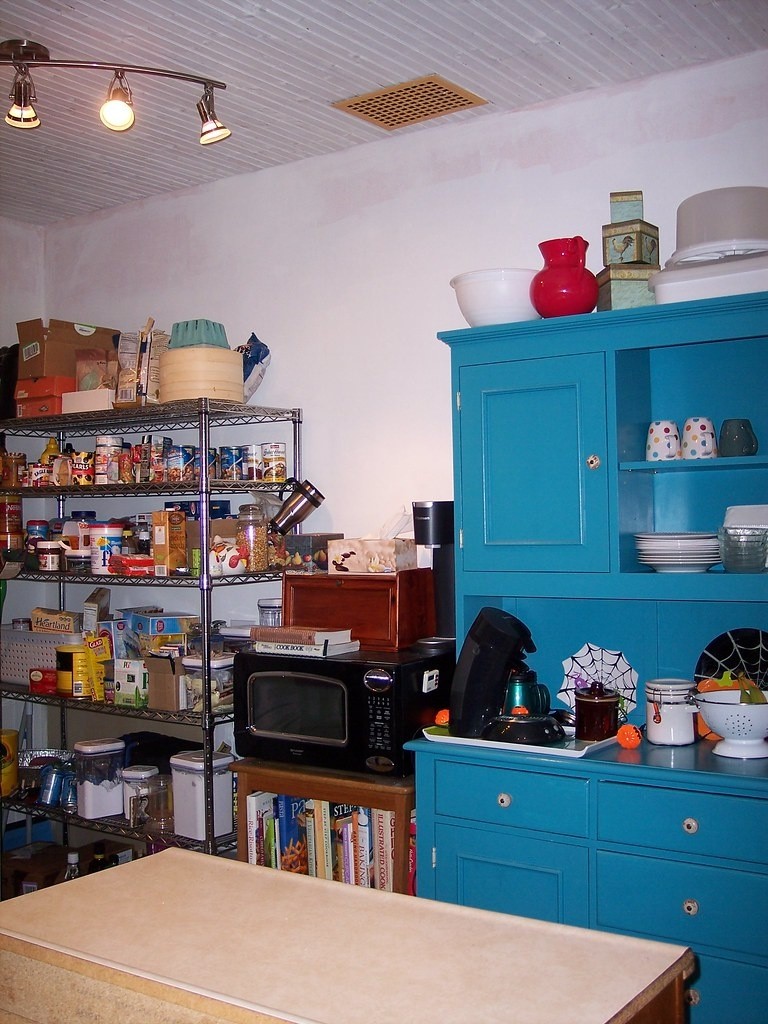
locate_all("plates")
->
[556,642,639,722]
[694,628,768,691]
[634,531,723,574]
[693,672,756,743]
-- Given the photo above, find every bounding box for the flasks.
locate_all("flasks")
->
[271,477,326,536]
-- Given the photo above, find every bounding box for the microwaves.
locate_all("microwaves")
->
[232,645,458,776]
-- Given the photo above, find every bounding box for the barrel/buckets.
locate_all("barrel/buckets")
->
[172,750,234,840]
[73,738,125,820]
[55,645,92,702]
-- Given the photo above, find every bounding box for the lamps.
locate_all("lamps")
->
[4,65,42,129]
[99,68,134,132]
[196,84,232,147]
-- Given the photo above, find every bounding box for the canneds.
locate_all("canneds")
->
[0,494,136,576]
[165,443,287,484]
[1,728,19,798]
[38,769,78,814]
[0,436,133,487]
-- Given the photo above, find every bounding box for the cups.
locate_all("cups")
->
[681,417,718,459]
[719,419,758,457]
[506,670,551,719]
[646,420,681,461]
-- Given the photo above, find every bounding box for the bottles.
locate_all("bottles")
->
[237,504,268,573]
[123,765,174,836]
[122,531,151,556]
[258,599,283,625]
[575,681,621,742]
[646,679,700,746]
[60,846,118,880]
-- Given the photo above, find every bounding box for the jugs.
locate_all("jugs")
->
[529,236,598,317]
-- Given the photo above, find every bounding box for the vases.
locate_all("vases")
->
[449,268,542,327]
[531,236,600,319]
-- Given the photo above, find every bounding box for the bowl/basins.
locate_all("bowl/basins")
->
[231,619,256,630]
[718,528,768,574]
[189,636,224,659]
[450,268,539,328]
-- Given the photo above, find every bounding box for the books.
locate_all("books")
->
[247,791,417,896]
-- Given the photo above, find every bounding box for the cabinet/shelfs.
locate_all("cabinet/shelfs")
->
[401,291,768,1024]
[0,396,302,903]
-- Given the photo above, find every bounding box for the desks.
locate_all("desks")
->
[228,757,416,896]
[0,847,696,1024]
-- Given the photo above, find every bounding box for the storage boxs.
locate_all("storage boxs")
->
[647,250,768,305]
[151,510,240,578]
[0,587,237,713]
[281,566,436,653]
[0,838,137,903]
[595,191,662,313]
[285,533,345,576]
[13,317,122,419]
